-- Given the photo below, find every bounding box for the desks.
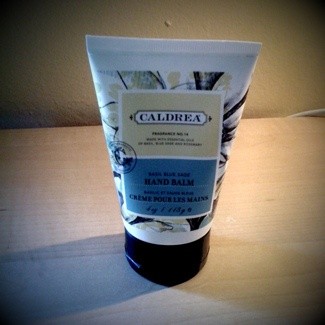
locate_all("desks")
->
[0,115,325,325]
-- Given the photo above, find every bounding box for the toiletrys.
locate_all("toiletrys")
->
[84,33,264,288]
